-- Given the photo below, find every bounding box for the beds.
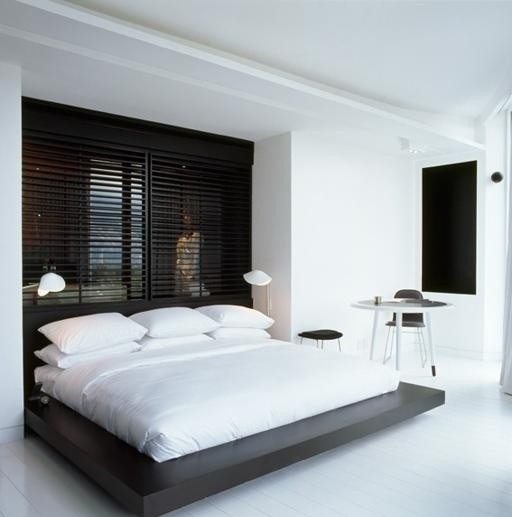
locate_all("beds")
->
[23,294,447,516]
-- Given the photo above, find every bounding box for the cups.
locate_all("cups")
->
[376,297,381,305]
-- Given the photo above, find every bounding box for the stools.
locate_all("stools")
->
[299,329,344,350]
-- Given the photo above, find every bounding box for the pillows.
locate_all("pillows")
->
[33,303,275,368]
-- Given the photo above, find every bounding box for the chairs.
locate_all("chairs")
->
[384,290,428,369]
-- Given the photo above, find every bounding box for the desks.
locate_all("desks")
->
[351,297,448,380]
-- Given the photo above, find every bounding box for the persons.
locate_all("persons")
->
[174,214,206,294]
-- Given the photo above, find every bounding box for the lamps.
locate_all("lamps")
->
[34,273,66,298]
[242,269,275,317]
